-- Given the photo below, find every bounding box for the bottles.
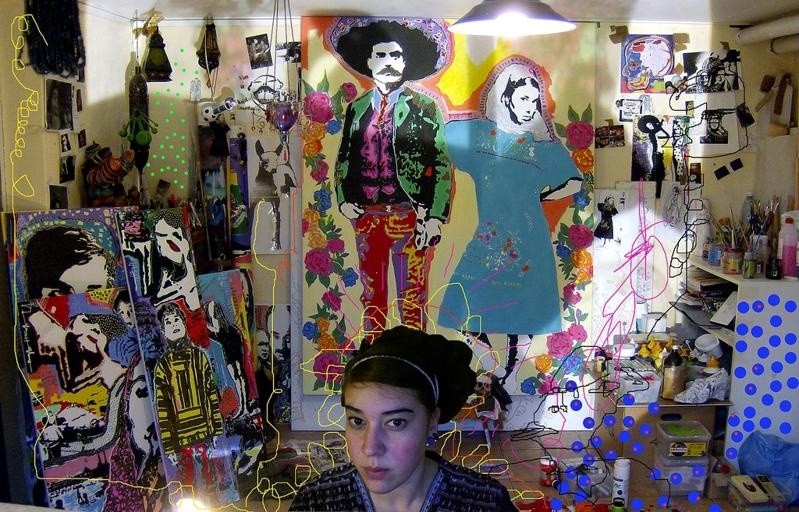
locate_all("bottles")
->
[727,248,741,275]
[610,457,630,508]
[743,192,754,224]
[742,252,755,279]
[721,249,728,273]
[778,218,798,279]
[662,345,685,400]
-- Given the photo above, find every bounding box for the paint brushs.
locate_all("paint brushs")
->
[749,196,781,253]
[707,205,749,249]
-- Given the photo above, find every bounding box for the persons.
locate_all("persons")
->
[286,325,514,511]
[322,16,454,346]
[152,303,224,511]
[436,54,584,394]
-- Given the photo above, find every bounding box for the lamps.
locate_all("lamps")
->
[446,0,576,40]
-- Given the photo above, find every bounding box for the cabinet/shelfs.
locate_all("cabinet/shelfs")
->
[690,250,795,474]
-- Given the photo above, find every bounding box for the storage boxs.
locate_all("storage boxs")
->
[592,379,716,473]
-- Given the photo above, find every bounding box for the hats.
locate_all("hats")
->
[326,17,454,84]
